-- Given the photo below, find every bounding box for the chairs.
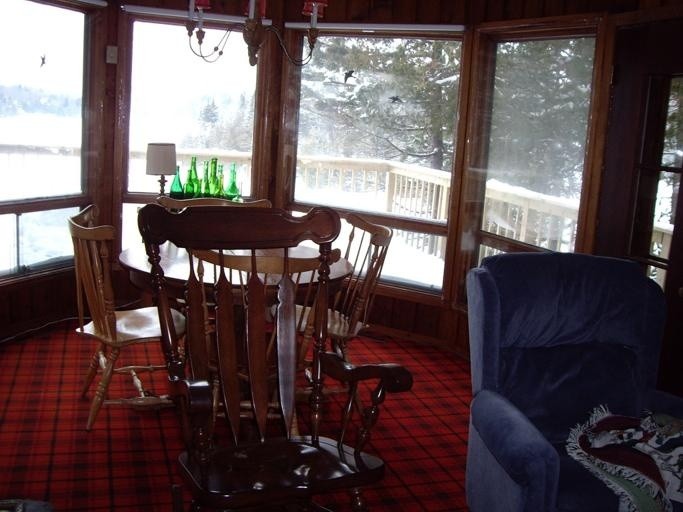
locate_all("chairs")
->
[465,251,682,511]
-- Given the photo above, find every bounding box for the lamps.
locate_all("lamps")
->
[145,143,178,197]
[185,0,328,67]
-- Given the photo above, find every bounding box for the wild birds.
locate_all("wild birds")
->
[40,55,47,67]
[388,96,404,105]
[343,70,357,81]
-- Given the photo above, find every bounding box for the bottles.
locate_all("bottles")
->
[168,155,243,206]
